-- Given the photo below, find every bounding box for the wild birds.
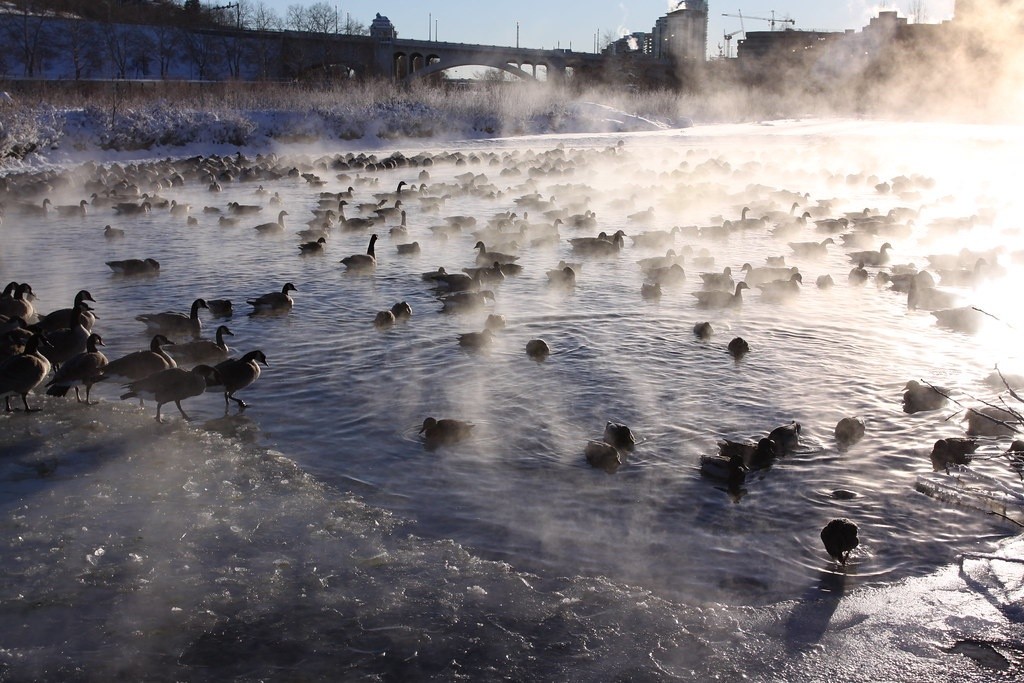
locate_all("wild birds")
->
[821,517,860,564]
[0,138,1024,494]
